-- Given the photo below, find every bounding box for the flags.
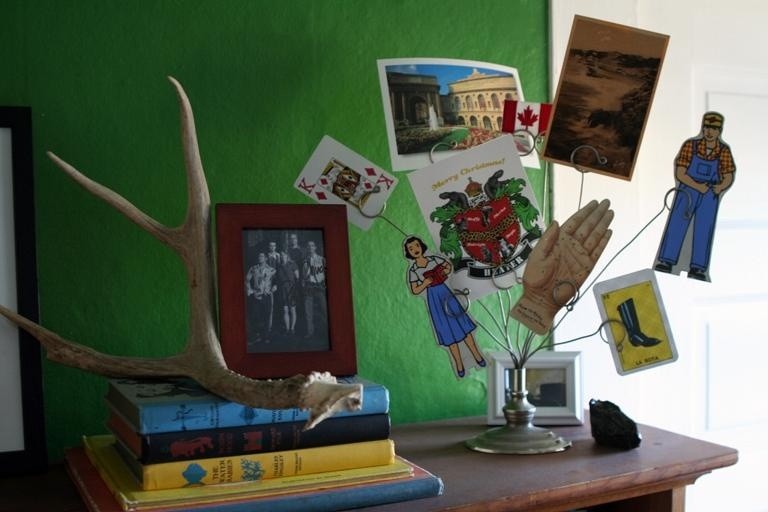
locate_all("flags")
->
[502,100,554,136]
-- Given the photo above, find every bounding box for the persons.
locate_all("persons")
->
[245,233,326,340]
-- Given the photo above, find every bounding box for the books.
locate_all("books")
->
[82,372,441,511]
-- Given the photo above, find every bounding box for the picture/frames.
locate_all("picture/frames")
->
[214,202,358,378]
[0,105,47,475]
[485,350,584,427]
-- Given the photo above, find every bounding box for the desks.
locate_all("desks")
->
[0,407,737,512]
